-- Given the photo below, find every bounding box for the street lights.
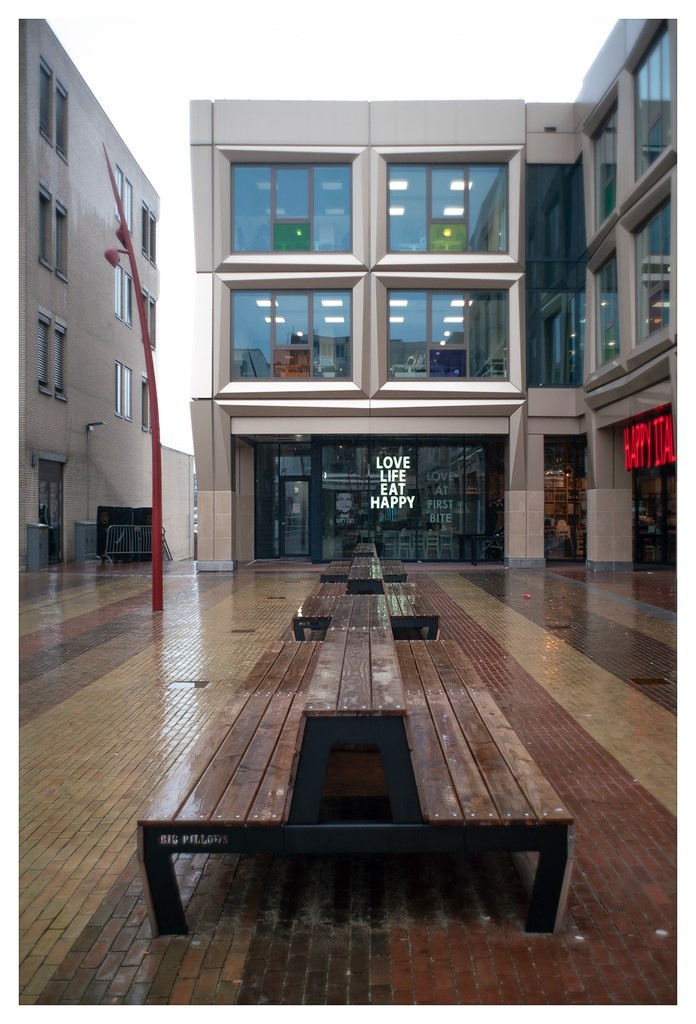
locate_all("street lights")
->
[103,247,163,610]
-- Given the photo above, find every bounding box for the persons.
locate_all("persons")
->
[336,493,354,525]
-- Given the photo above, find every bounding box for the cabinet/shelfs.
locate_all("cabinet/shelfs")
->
[544,472,581,529]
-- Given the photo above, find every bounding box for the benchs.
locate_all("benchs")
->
[138,543,574,935]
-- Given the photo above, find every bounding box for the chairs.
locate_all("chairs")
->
[358,530,453,558]
